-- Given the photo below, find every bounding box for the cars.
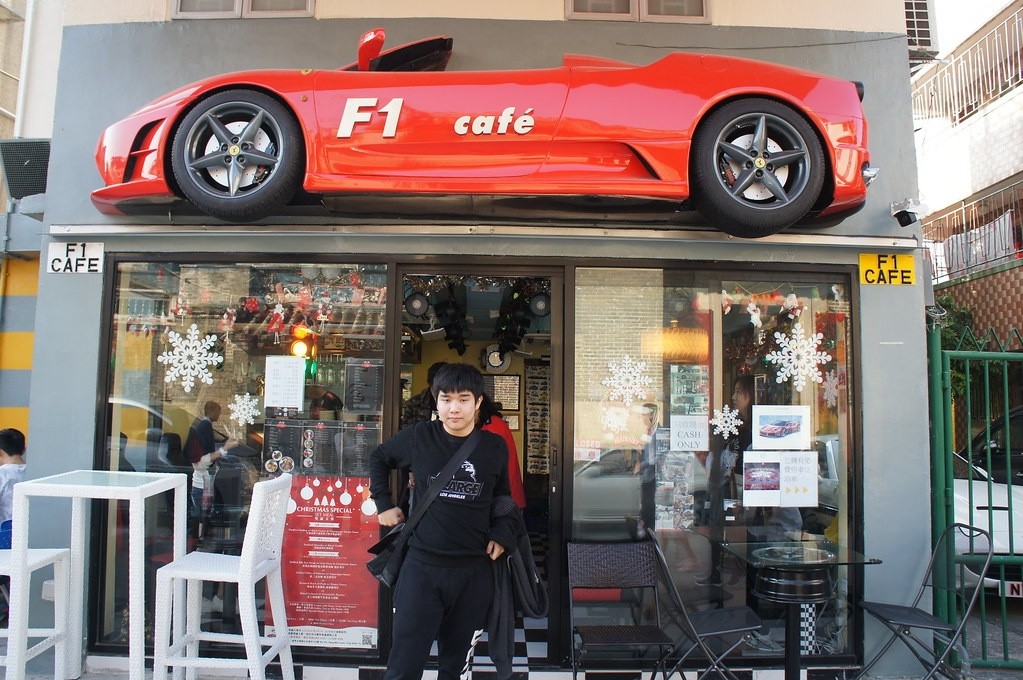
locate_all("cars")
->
[570,447,713,530]
[956,401,1023,480]
[107,395,261,518]
[744,435,838,533]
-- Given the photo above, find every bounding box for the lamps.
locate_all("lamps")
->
[891,197,920,227]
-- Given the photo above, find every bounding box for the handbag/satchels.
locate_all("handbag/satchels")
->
[366,523,414,591]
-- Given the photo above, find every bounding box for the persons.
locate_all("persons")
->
[724,375,771,476]
[0,428,26,620]
[309,399,335,420]
[404,363,527,518]
[634,404,803,586]
[370,363,520,680]
[184,401,221,473]
[191,380,241,615]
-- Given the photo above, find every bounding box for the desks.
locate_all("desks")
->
[721,540,883,680]
[4,470,189,680]
[686,519,827,637]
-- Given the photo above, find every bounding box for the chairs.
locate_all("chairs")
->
[153,476,296,680]
[575,520,644,659]
[0,549,71,680]
[566,540,677,680]
[646,527,763,680]
[853,524,994,680]
[625,517,734,656]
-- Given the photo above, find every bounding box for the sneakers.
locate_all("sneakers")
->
[746,631,785,652]
[726,573,746,587]
[694,574,722,587]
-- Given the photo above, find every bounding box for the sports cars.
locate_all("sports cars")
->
[951,449,1023,599]
[90,25,879,238]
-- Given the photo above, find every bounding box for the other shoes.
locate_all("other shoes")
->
[202,595,240,614]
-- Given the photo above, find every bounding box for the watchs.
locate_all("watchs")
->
[219,446,228,457]
